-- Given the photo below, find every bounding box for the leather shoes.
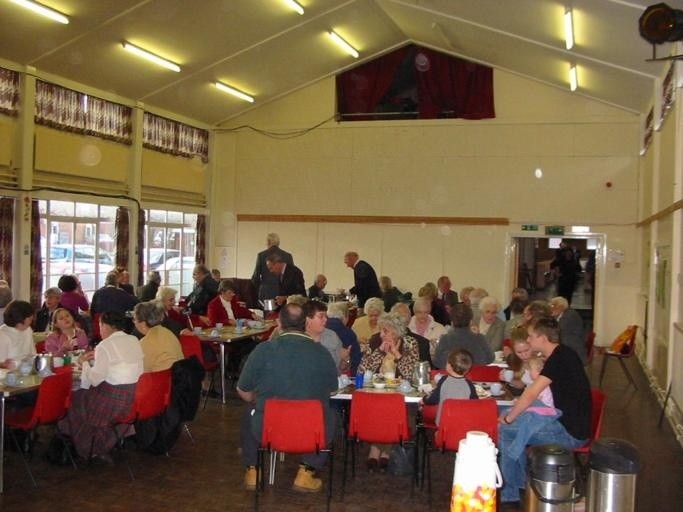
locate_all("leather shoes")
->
[367,457,388,469]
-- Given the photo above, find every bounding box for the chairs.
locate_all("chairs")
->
[597,323,641,391]
[89,369,173,482]
[420,397,498,509]
[254,398,344,509]
[345,387,422,498]
[4,371,77,488]
[136,355,202,456]
[570,385,606,483]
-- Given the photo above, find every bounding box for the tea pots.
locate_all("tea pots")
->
[258,298,278,312]
[410,360,431,392]
[34,350,55,376]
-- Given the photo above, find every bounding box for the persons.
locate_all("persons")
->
[1,266,222,468]
[180,231,306,377]
[237,239,596,512]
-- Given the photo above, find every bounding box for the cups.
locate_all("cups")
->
[494,351,504,362]
[490,383,501,394]
[355,373,364,390]
[194,323,223,335]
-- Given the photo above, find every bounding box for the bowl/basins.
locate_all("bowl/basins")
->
[5,365,32,385]
[248,320,256,329]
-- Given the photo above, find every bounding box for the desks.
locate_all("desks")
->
[188,318,275,403]
[1,350,95,398]
[327,338,520,489]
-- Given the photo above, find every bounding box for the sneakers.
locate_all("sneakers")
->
[294,465,322,491]
[244,466,257,490]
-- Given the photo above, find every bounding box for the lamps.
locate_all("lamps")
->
[120,40,180,72]
[8,1,71,26]
[568,63,577,92]
[328,31,360,59]
[216,82,254,103]
[563,7,574,51]
[290,1,303,14]
[638,1,682,63]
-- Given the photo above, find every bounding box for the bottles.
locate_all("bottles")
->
[331,294,336,303]
[447,430,502,511]
[523,444,584,511]
[586,438,647,511]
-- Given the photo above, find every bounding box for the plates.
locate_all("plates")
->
[485,390,507,397]
[371,376,408,388]
